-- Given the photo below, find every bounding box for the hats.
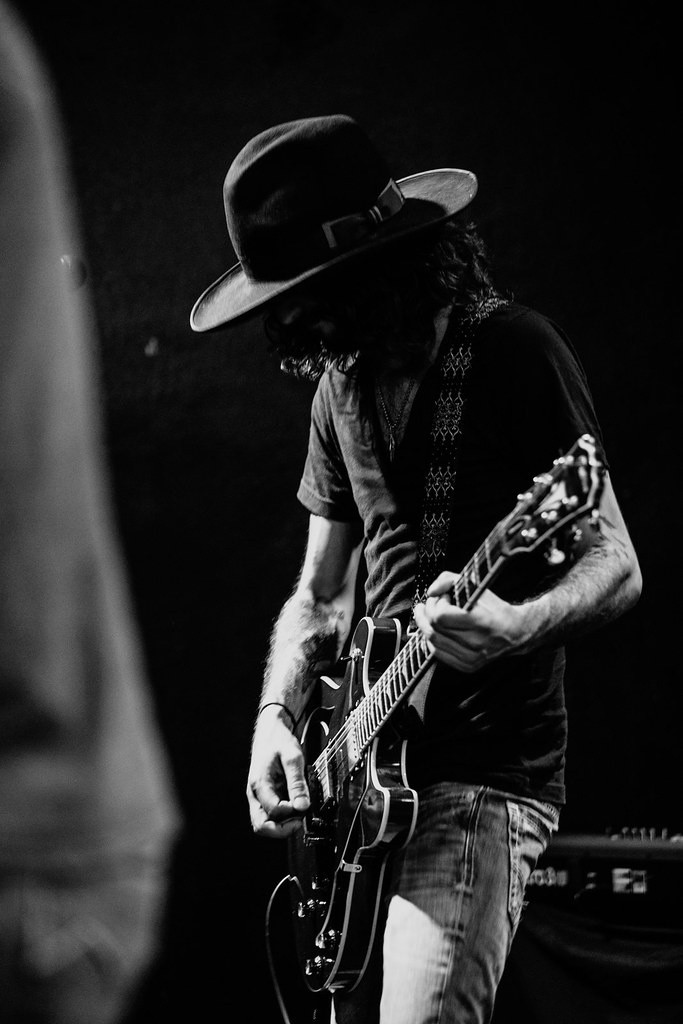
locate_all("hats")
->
[187,114,481,333]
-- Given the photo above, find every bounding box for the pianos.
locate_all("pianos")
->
[523,824,683,930]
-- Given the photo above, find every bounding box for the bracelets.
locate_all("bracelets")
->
[259,702,297,735]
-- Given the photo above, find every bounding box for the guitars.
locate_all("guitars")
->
[287,430,606,996]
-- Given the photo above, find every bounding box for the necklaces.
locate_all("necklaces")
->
[374,374,416,462]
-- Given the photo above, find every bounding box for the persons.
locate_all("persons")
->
[187,116,643,1024]
[0,18,176,1024]
